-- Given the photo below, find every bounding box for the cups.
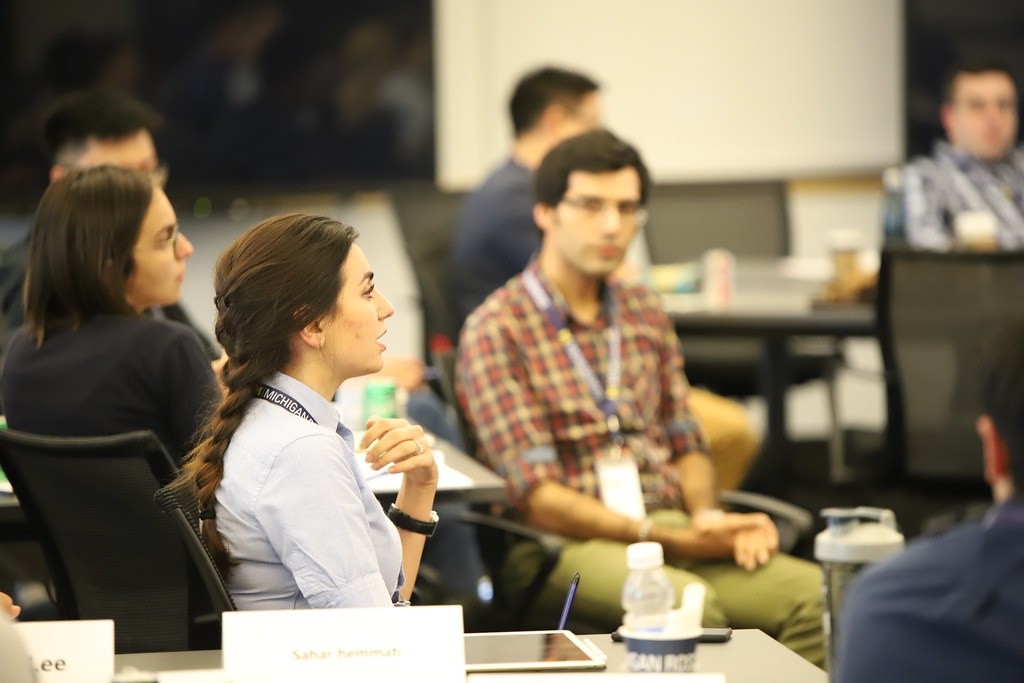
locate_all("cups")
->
[814,507,905,683]
[617,624,704,674]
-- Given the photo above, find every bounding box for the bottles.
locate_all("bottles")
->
[622,541,676,634]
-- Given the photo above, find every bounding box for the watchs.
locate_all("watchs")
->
[388,503,439,538]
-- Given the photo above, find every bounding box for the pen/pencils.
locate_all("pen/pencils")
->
[559,572,580,630]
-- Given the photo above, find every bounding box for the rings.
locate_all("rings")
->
[413,440,424,456]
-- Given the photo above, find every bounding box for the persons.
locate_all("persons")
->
[879,51,1024,253]
[450,125,827,670]
[0,163,220,468]
[1,88,219,363]
[828,351,1024,683]
[182,213,439,614]
[457,66,759,490]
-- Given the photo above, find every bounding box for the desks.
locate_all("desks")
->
[114,629,831,683]
[353,428,507,502]
[668,285,874,336]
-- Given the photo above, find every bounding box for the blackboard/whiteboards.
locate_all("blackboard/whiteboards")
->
[432,2,907,193]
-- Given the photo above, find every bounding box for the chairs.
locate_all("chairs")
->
[642,179,848,483]
[152,484,237,612]
[404,353,812,628]
[0,428,202,652]
[876,247,1023,512]
[388,180,472,402]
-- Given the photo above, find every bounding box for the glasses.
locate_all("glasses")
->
[131,219,182,254]
[132,161,169,192]
[559,195,650,230]
